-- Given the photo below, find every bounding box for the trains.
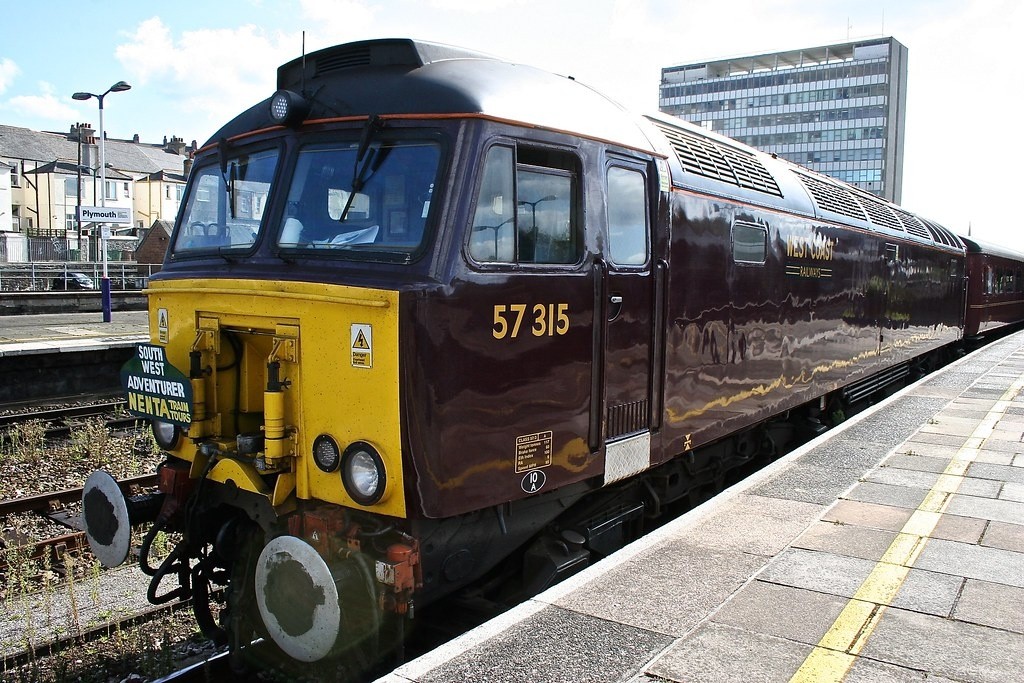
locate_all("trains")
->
[75,36,1024,683]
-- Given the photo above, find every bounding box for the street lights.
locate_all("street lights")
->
[71,79,132,323]
[76,160,115,264]
[68,122,96,261]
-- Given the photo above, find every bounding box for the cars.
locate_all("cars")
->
[51,269,95,292]
